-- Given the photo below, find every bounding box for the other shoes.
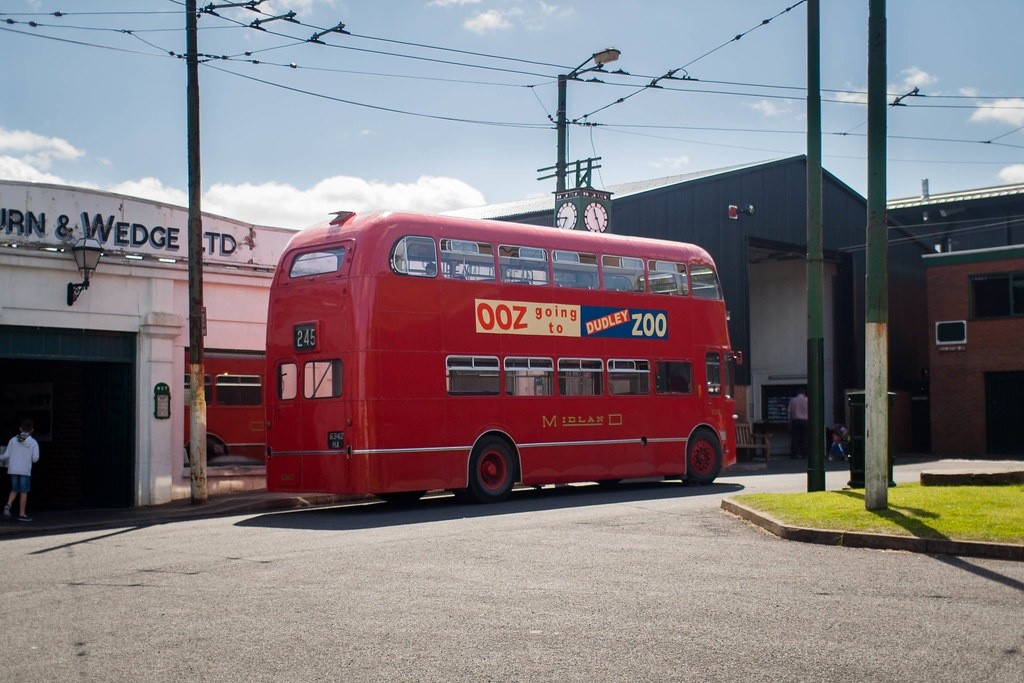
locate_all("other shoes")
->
[17,515,33,521]
[4,505,10,517]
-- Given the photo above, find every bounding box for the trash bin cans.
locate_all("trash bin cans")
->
[846,391,897,489]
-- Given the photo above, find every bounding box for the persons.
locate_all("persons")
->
[0,418,39,521]
[426,263,436,275]
[787,387,808,460]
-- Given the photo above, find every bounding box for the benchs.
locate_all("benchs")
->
[735,421,772,462]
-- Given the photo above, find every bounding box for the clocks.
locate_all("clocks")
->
[583,202,608,233]
[555,201,578,230]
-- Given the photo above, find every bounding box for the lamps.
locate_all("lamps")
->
[67,237,105,307]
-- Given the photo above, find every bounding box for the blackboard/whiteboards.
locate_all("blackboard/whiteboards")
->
[760,383,808,420]
